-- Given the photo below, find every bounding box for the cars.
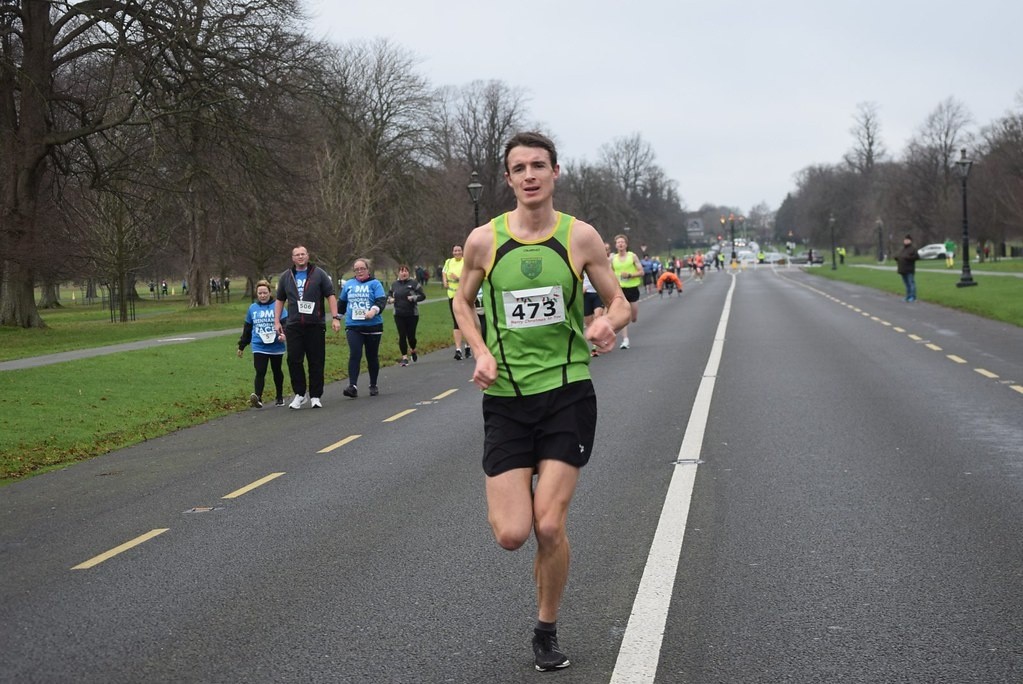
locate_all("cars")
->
[791,250,825,263]
[916,243,955,264]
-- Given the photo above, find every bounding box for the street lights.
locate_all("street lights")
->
[466,170,485,230]
[719,212,744,267]
[640,243,648,258]
[876,218,886,264]
[952,148,978,288]
[666,238,675,258]
[828,213,838,270]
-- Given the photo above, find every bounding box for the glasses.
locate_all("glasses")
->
[354,267,367,273]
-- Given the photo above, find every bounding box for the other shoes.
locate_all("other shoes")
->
[903,297,918,303]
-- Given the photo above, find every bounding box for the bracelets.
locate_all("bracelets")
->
[629,274,631,278]
[333,316,339,320]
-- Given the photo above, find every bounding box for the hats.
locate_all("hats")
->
[905,234,912,242]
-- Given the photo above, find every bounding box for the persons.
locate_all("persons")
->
[895,234,919,302]
[182,278,187,293]
[808,250,813,265]
[437,265,443,281]
[453,132,632,671]
[984,245,990,258]
[148,280,157,297]
[688,250,705,281]
[706,253,738,271]
[337,257,387,400]
[582,235,645,357]
[237,279,288,409]
[162,279,168,296]
[274,245,340,410]
[640,253,684,294]
[837,245,847,264]
[210,276,231,292]
[944,237,957,268]
[758,251,764,264]
[442,244,487,361]
[387,265,426,367]
[415,266,429,286]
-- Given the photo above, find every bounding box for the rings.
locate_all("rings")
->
[602,341,608,346]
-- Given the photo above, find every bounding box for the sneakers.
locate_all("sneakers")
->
[250,393,263,408]
[465,348,472,359]
[591,349,600,357]
[454,351,462,360]
[275,398,284,406]
[369,384,379,396]
[343,385,358,398]
[620,341,630,349]
[289,394,307,409]
[401,359,409,366]
[532,634,570,672]
[311,397,322,408]
[411,351,418,363]
